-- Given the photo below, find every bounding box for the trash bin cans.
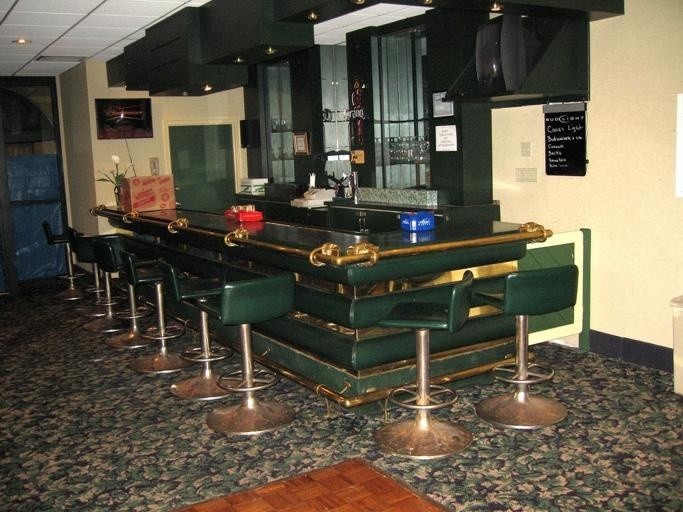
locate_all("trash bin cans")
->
[668,294,682,394]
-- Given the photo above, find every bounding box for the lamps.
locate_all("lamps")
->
[327,149,352,200]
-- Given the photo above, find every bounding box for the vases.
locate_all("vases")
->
[114,185,120,208]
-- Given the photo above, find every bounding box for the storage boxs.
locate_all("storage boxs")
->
[120,173,176,214]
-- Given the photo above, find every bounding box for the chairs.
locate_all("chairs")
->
[118,249,191,375]
[201,273,298,436]
[474,263,580,430]
[155,257,232,401]
[42,219,91,301]
[74,234,118,317]
[91,239,152,351]
[376,269,477,461]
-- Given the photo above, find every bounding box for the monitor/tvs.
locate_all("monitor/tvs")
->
[472,13,527,96]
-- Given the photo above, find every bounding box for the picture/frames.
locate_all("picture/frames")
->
[290,130,310,156]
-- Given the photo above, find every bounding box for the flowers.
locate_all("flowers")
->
[94,155,132,187]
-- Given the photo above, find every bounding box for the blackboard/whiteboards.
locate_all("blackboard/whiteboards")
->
[544,111,586,177]
